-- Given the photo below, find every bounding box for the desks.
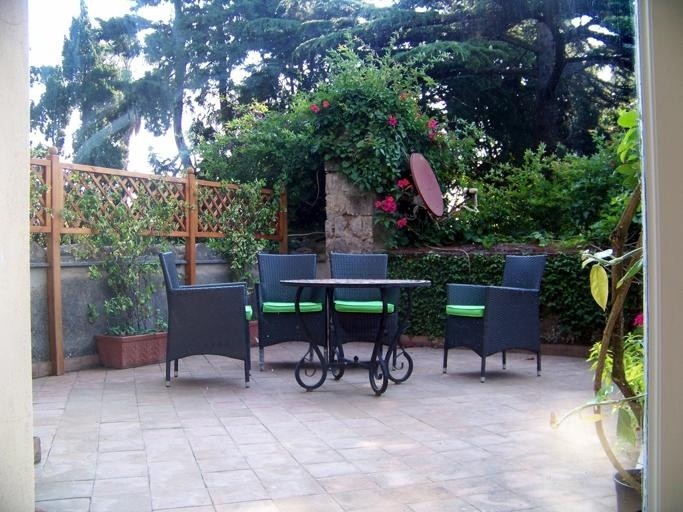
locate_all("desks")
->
[276,277,432,395]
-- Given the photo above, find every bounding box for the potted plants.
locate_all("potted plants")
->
[202,192,287,346]
[586,330,647,510]
[54,188,195,370]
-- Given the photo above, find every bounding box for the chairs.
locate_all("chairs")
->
[158,249,393,386]
[442,253,547,382]
